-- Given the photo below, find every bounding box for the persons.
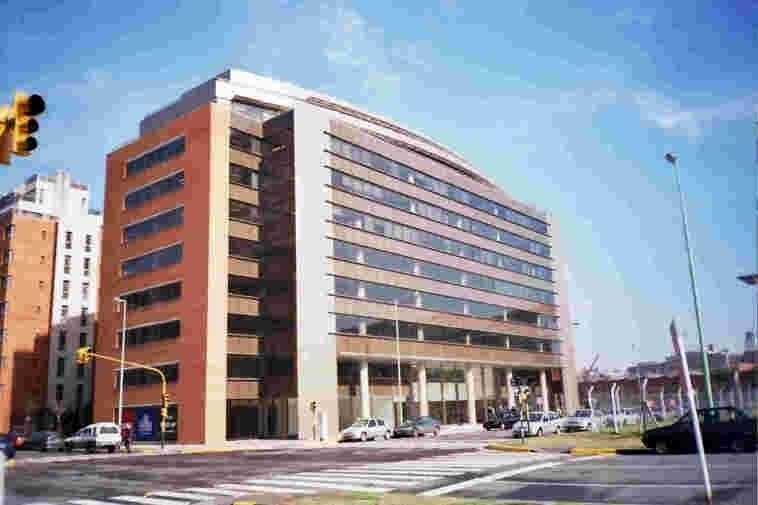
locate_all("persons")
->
[6,424,16,459]
[123,423,132,452]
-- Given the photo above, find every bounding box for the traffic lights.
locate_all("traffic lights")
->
[77,346,93,365]
[9,90,45,157]
[161,393,170,411]
[511,375,520,387]
[308,400,316,412]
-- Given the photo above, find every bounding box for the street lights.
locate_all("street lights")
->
[560,321,579,416]
[113,297,128,448]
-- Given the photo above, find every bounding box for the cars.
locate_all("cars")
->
[337,416,394,443]
[394,414,442,440]
[0,421,122,460]
[641,406,757,455]
[484,407,757,437]
